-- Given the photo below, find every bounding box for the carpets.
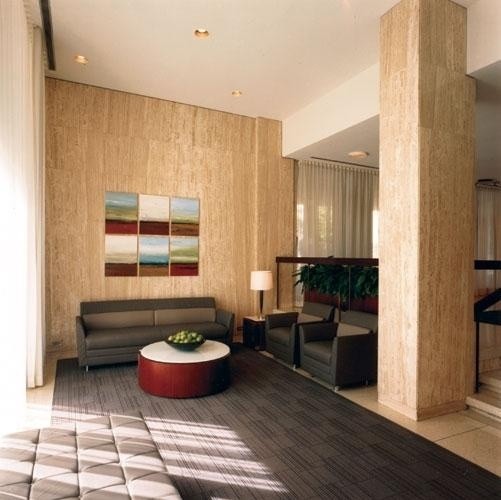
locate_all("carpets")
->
[52,341,501,500]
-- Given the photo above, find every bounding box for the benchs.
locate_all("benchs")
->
[0,411,183,500]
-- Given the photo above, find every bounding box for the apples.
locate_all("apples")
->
[168,330,204,344]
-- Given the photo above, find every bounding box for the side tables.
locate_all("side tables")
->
[243,316,266,352]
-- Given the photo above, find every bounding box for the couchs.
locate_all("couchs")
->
[76,298,235,372]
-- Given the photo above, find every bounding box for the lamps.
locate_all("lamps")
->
[249,270,274,321]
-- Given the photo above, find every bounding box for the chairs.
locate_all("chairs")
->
[298,310,378,391]
[264,301,336,369]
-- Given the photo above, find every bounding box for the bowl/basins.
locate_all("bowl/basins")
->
[165,333,207,351]
